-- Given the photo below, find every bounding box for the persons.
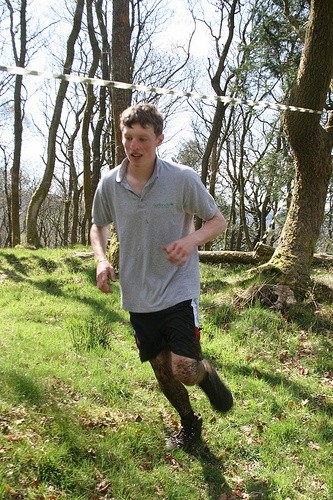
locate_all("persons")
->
[89,104,234,449]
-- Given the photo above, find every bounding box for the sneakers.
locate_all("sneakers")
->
[200,363,234,414]
[166,415,203,451]
